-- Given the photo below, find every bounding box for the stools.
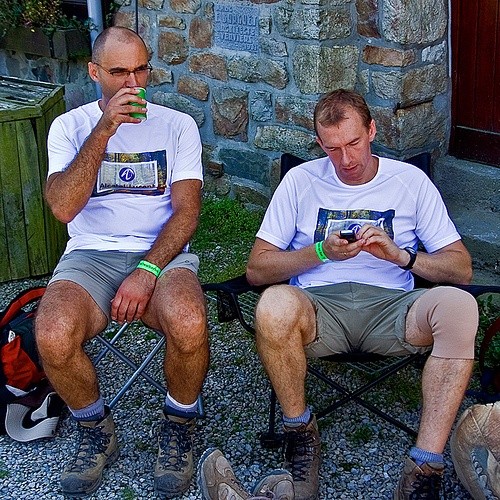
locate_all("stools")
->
[91,322,203,416]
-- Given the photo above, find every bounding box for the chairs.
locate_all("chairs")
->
[201,152,500,448]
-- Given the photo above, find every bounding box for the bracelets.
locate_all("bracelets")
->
[136,260,161,276]
[315,241,330,263]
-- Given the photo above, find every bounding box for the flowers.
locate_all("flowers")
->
[0,0,99,41]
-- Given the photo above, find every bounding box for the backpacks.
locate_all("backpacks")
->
[0,286,45,396]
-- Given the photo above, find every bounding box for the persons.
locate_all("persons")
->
[247,89,479,500]
[34,27,208,497]
[197,447,296,500]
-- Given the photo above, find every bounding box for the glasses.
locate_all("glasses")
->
[93,61,153,79]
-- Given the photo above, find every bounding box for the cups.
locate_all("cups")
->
[128,87,147,122]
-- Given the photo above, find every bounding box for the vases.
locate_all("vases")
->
[3,26,93,58]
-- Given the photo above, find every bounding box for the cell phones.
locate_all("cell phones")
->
[339,230,357,244]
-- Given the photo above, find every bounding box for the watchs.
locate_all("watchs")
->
[398,247,417,270]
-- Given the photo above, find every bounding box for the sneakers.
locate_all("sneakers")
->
[154,404,198,495]
[61,405,121,496]
[281,414,321,500]
[252,469,296,500]
[393,456,445,500]
[198,448,271,500]
